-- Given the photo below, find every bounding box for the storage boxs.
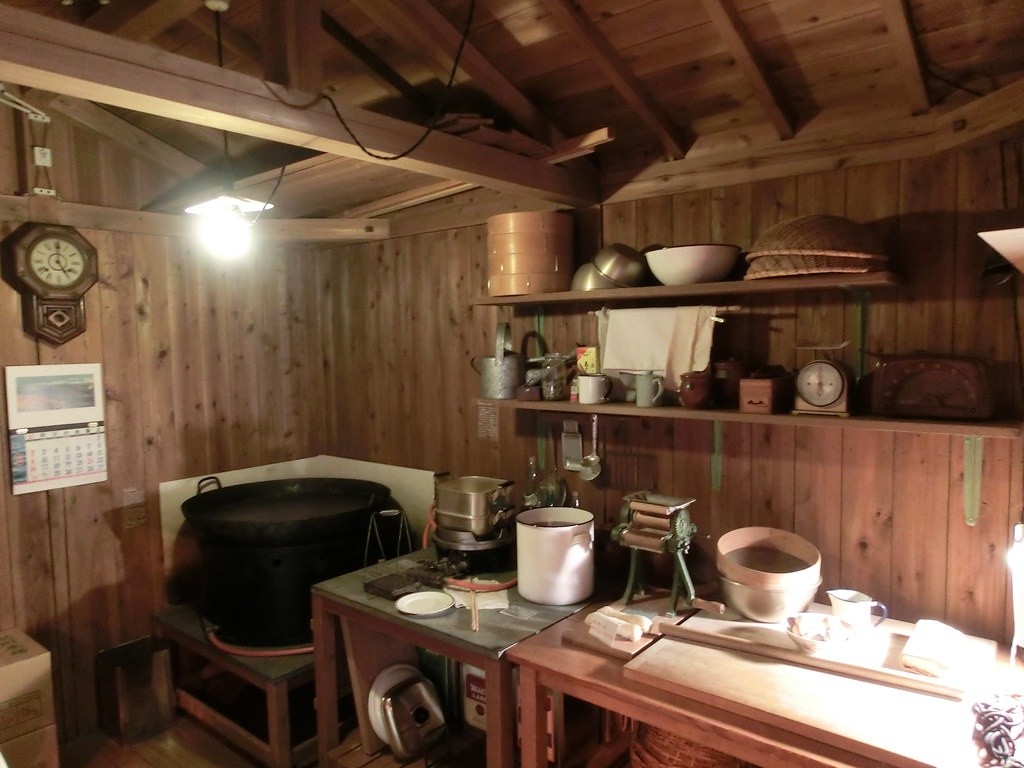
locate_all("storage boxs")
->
[0,627,66,768]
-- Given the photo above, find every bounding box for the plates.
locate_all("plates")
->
[394,591,455,615]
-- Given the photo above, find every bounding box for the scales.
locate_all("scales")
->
[789,336,853,419]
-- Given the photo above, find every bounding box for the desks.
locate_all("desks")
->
[149,609,342,768]
[503,566,1024,767]
[312,546,618,767]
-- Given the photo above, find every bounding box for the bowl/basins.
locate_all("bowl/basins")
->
[571,263,619,291]
[785,612,853,654]
[591,243,649,288]
[645,244,742,285]
[719,573,823,623]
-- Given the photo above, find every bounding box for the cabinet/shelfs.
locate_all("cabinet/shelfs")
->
[472,272,1024,446]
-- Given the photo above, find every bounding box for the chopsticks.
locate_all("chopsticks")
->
[471,591,479,632]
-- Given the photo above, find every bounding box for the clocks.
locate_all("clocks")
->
[7,223,97,346]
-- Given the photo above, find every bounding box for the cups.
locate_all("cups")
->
[715,357,746,409]
[578,373,608,405]
[636,370,664,408]
[680,371,712,410]
[827,590,887,626]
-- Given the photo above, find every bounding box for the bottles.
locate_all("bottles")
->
[570,379,578,403]
[520,457,544,511]
[542,353,566,401]
[571,491,583,509]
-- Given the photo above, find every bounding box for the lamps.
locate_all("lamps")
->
[185,0,274,265]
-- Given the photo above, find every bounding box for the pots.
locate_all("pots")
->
[434,472,515,537]
[181,476,391,546]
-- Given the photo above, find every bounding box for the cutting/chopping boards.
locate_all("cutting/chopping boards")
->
[623,602,997,768]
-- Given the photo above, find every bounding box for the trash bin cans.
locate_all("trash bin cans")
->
[97,635,176,746]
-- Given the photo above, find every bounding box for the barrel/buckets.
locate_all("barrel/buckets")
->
[480,322,520,400]
[516,506,595,605]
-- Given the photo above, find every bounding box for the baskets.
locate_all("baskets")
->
[743,254,886,280]
[743,215,886,257]
